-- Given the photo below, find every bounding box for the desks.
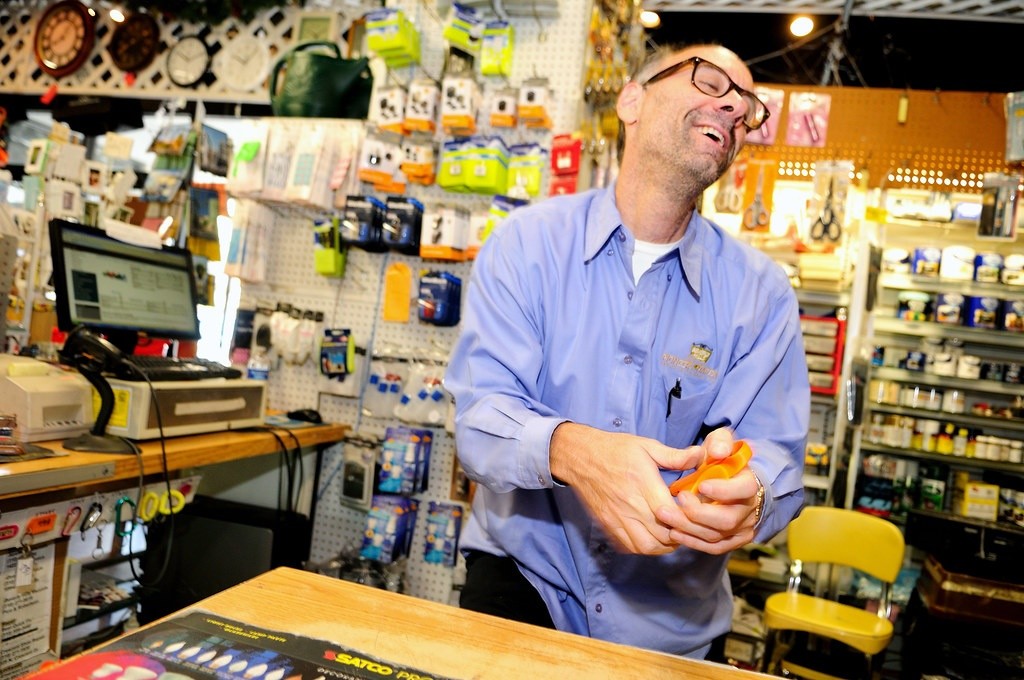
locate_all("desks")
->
[0,411,351,635]
[15,564,800,680]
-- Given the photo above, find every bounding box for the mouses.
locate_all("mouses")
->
[287,408,323,423]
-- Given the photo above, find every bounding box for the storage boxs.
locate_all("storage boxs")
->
[222,5,549,325]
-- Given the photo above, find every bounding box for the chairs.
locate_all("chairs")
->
[762,505,904,680]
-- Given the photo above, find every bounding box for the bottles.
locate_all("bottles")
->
[856,452,917,521]
[872,415,1024,461]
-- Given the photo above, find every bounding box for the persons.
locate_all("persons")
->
[448,46,814,665]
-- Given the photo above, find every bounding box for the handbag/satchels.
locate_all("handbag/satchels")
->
[270,41,374,120]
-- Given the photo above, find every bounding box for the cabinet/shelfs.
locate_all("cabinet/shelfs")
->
[745,178,1024,679]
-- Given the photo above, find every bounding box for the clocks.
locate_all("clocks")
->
[293,12,338,45]
[218,34,272,92]
[165,36,211,88]
[107,13,159,70]
[34,0,96,79]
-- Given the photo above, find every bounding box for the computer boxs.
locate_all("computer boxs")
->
[136,492,312,625]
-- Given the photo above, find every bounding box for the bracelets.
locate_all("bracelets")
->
[754,468,765,539]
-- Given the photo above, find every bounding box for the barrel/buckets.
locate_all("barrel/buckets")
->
[872,338,1024,385]
[870,381,966,415]
[900,291,1024,333]
[883,245,1023,286]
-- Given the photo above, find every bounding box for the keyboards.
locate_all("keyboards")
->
[116,354,231,382]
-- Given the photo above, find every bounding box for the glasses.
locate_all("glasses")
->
[643,57,770,134]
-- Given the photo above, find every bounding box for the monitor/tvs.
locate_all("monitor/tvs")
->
[48,217,201,373]
